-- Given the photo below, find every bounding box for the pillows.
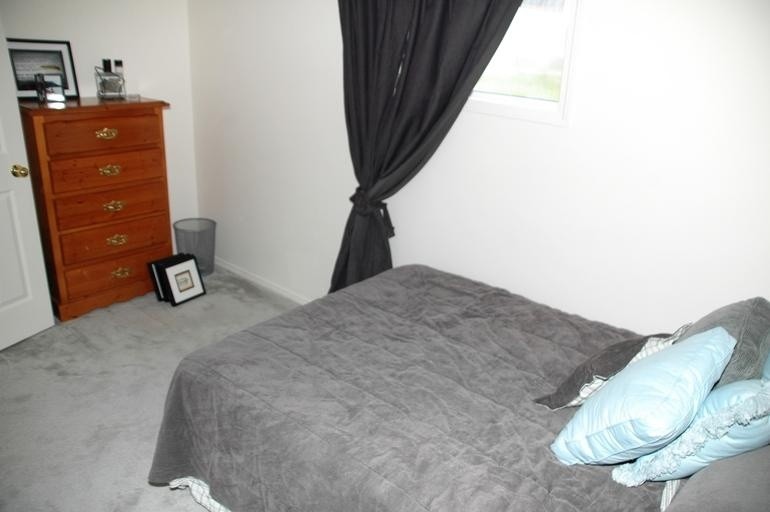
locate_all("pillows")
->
[550,326,737,467]
[532,334,694,411]
[664,445,769,511]
[673,297,769,392]
[610,356,769,488]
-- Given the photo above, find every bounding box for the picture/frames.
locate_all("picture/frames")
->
[164,254,205,305]
[148,263,166,301]
[7,39,76,99]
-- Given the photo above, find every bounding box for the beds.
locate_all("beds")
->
[147,265,768,512]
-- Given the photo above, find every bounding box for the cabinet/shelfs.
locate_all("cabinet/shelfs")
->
[19,96,175,321]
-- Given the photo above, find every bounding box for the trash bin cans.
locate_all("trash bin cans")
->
[173,218,216,275]
